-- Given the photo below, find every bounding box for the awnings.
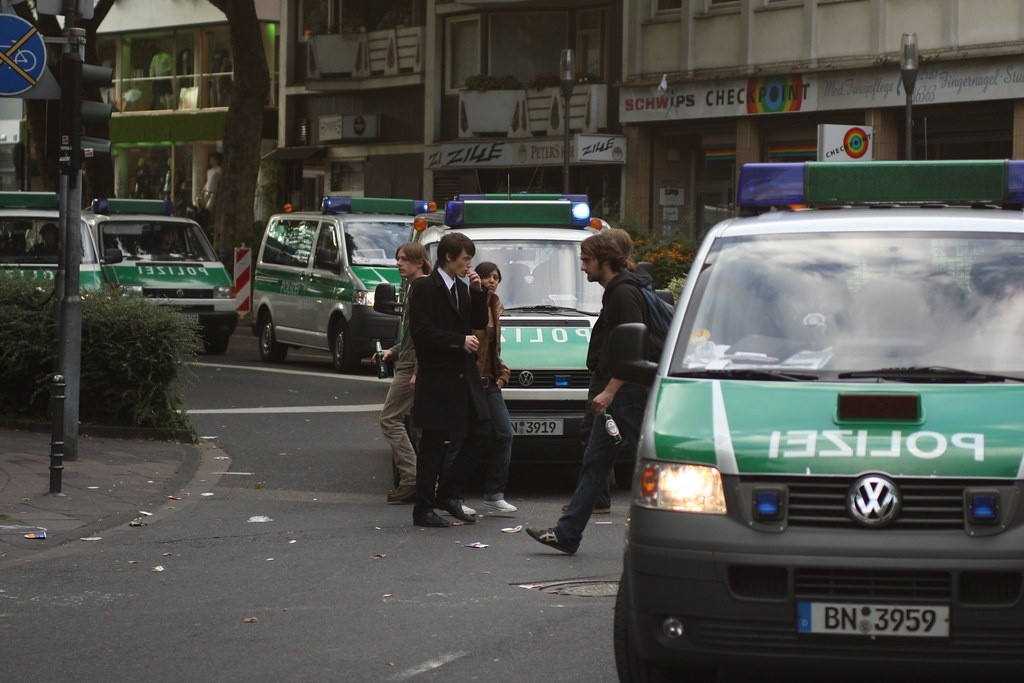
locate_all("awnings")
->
[259,147,327,161]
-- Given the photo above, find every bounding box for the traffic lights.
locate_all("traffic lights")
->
[75,63,116,123]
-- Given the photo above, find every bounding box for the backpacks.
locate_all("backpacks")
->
[602,279,675,364]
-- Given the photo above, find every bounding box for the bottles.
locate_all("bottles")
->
[375,338,388,379]
[601,410,622,445]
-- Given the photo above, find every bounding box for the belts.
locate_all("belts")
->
[481,376,496,388]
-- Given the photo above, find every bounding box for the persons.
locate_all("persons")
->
[152,228,180,261]
[371,232,518,528]
[525,228,676,557]
[29,222,61,259]
[100,40,231,227]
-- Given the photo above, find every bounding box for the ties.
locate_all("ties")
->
[450,283,457,309]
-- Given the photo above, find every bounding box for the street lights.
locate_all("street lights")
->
[558,47,578,194]
[898,31,919,161]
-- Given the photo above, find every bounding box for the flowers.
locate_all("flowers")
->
[305,9,413,47]
[459,73,601,93]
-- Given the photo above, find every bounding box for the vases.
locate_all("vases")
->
[458,83,607,139]
[306,26,426,79]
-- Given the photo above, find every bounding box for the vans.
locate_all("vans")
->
[248,213,421,377]
[0,208,239,359]
[373,223,678,498]
[599,211,1023,683]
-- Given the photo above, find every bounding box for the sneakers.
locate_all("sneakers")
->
[525,527,575,554]
[443,505,476,515]
[482,499,517,512]
[413,510,449,527]
[435,490,475,523]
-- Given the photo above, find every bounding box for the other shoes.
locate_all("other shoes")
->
[562,504,611,514]
[387,484,416,502]
